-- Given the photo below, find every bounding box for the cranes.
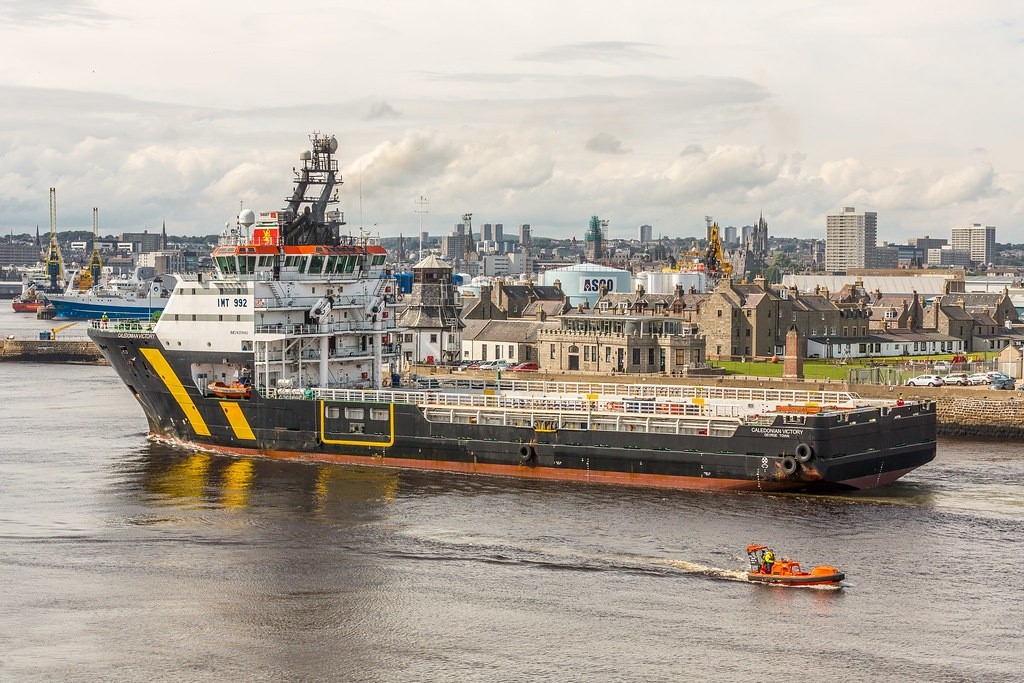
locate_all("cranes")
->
[45,187,65,293]
[84,207,102,285]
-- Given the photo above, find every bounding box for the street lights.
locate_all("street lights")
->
[413,194,429,262]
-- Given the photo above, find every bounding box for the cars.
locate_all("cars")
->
[1017,383,1024,390]
[987,372,1002,378]
[988,378,1015,390]
[437,359,538,372]
[943,373,967,385]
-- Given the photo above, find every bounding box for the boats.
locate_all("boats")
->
[85,130,937,495]
[747,545,845,588]
[12,284,48,311]
[42,267,171,321]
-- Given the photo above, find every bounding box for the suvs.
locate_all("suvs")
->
[968,374,994,385]
[907,375,943,387]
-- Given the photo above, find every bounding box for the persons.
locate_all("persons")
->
[494,367,502,390]
[897,393,903,400]
[611,366,615,376]
[763,549,775,574]
